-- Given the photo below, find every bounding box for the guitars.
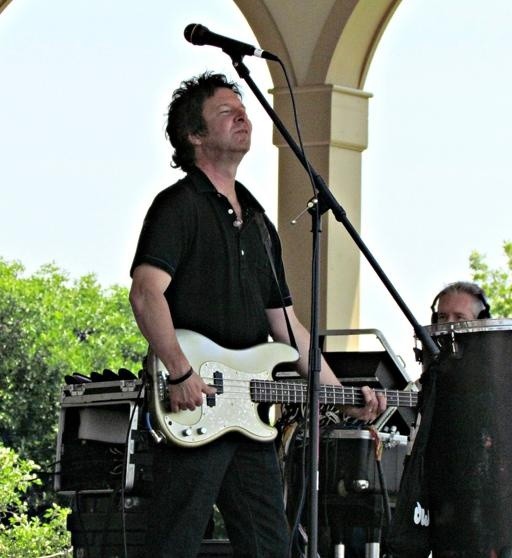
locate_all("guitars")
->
[147,329,420,447]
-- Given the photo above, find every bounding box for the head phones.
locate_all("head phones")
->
[430,286,491,324]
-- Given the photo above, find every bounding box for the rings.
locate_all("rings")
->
[365,405,374,415]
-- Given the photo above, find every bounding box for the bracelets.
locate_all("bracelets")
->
[162,365,194,388]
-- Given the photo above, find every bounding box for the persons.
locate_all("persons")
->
[134,70,387,556]
[430,282,494,327]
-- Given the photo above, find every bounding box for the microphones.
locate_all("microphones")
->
[184,24,278,62]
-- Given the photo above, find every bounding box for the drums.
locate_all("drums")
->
[419,319,512,558]
